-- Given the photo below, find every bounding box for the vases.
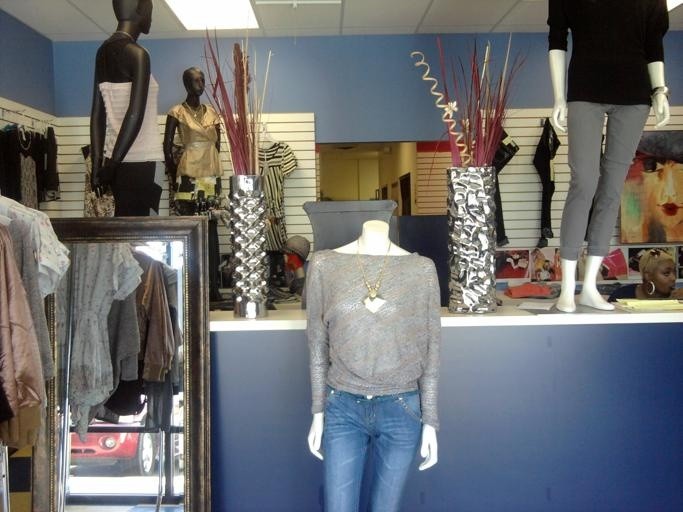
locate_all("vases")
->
[227,175,269,318]
[444,166,497,314]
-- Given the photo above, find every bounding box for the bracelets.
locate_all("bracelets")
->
[649,86,669,99]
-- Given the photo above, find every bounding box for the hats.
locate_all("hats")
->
[283,234,312,261]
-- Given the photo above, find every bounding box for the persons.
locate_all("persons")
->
[553,249,561,280]
[87,0,167,218]
[532,249,545,282]
[162,65,222,300]
[608,246,682,303]
[546,0,670,314]
[304,220,445,512]
[495,249,523,276]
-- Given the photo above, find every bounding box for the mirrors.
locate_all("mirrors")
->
[30,216,212,511]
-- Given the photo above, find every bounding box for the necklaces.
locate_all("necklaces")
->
[112,31,135,43]
[355,235,392,315]
[184,102,203,119]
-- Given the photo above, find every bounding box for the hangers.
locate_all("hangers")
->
[255,123,281,147]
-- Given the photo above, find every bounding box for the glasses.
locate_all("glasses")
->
[642,247,666,272]
